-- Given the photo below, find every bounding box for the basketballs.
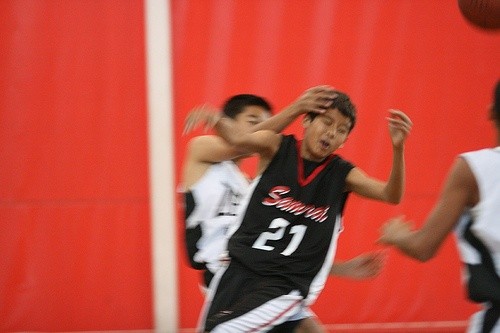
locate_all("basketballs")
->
[456,0,499,30]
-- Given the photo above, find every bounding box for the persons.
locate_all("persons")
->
[180,91,412,333]
[184,85,386,299]
[378,79,500,332]
